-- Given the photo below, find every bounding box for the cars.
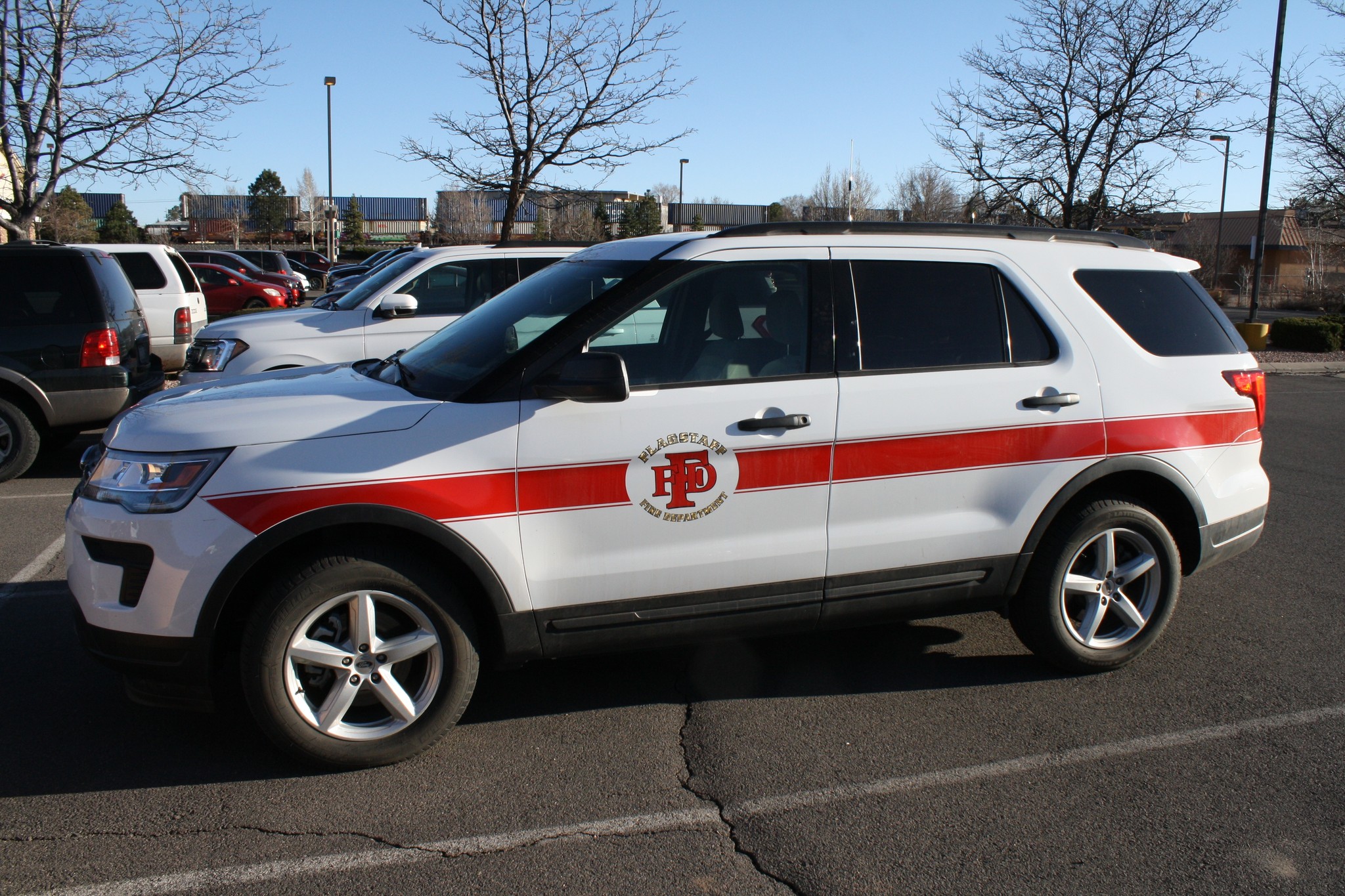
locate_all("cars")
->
[186,262,293,315]
[286,258,328,291]
[292,271,310,291]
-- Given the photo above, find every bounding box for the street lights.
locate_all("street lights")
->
[677,158,689,233]
[324,76,336,269]
[1209,134,1231,290]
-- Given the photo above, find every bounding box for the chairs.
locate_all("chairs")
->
[680,296,750,381]
[753,290,810,375]
[468,271,492,310]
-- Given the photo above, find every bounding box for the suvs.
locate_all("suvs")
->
[0,239,167,482]
[175,239,770,388]
[210,249,294,277]
[34,244,208,372]
[64,222,1274,777]
[282,251,351,272]
[175,249,306,308]
